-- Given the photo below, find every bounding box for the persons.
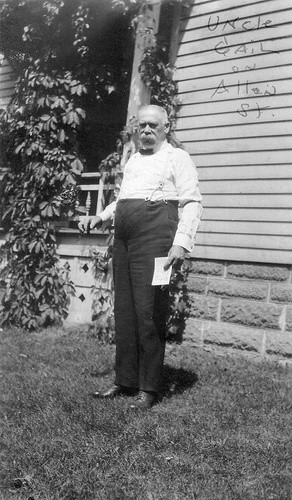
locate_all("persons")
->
[78,104,204,412]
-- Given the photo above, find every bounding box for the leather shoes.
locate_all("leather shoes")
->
[93,381,137,398]
[128,390,156,410]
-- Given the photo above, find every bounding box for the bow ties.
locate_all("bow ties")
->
[139,148,154,155]
